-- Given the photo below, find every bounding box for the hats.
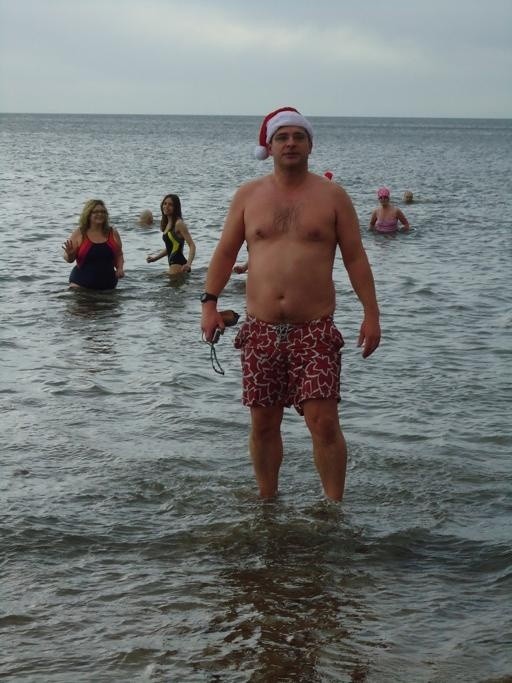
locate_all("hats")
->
[378,188,390,199]
[254,107,313,160]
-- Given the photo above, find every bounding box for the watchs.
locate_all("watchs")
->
[201,293,218,303]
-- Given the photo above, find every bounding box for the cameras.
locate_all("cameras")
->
[211,327,221,343]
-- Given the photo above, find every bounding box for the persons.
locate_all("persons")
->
[404,191,413,204]
[368,188,409,233]
[234,262,248,274]
[201,107,380,499]
[62,201,124,290]
[141,208,153,225]
[146,195,195,275]
